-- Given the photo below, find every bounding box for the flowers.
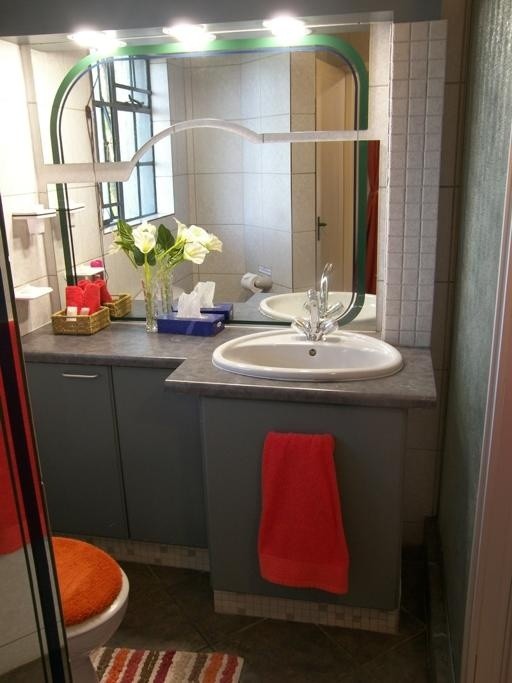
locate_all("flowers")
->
[113,217,224,299]
[107,213,209,312]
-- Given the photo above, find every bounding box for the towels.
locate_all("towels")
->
[256,429,351,598]
[77,278,114,306]
[64,284,100,319]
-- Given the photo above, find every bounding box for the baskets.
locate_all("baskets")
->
[50,306,110,335]
[102,294,132,320]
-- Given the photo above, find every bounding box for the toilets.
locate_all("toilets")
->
[48,529,164,683]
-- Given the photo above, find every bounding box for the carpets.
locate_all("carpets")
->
[87,646,244,682]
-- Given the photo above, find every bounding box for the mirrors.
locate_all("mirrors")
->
[16,19,396,333]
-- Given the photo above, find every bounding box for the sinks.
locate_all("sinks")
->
[212,330,404,381]
[259,291,377,330]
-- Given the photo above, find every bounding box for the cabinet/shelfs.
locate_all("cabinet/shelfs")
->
[198,394,408,615]
[23,361,209,569]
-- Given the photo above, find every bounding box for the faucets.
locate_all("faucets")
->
[320,262,337,310]
[307,288,319,331]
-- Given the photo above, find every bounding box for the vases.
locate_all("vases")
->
[157,268,176,318]
[141,277,162,335]
